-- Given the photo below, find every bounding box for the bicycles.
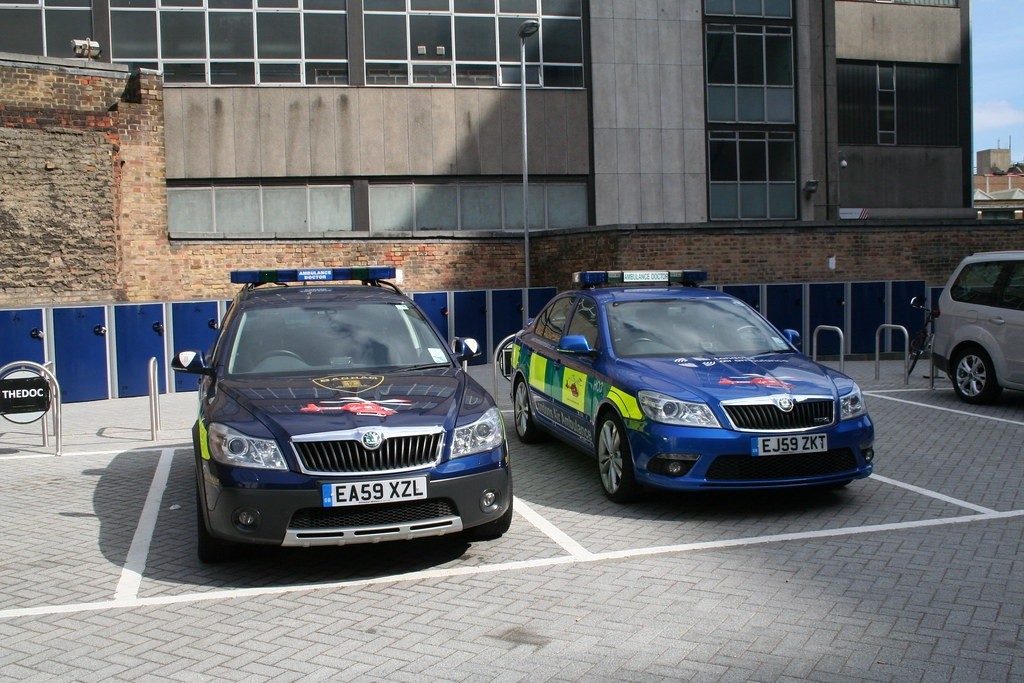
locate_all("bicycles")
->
[908,298,937,375]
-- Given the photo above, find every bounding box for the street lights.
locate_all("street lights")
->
[518,20,541,287]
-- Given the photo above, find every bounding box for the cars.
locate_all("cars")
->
[171,266,513,566]
[933,250,1024,405]
[511,268,878,504]
